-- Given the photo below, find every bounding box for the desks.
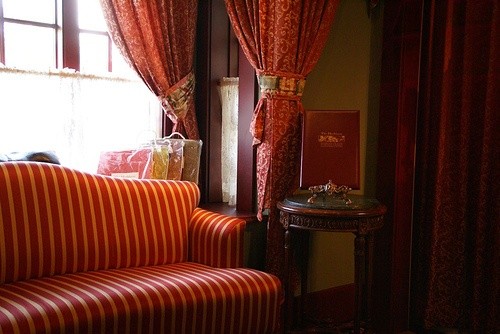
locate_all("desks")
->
[276,191,387,334]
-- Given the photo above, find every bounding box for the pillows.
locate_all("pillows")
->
[153,138,202,185]
[97,147,152,179]
[146,140,184,180]
[136,144,170,181]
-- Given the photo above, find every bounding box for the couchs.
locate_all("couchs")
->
[0,160,284,333]
